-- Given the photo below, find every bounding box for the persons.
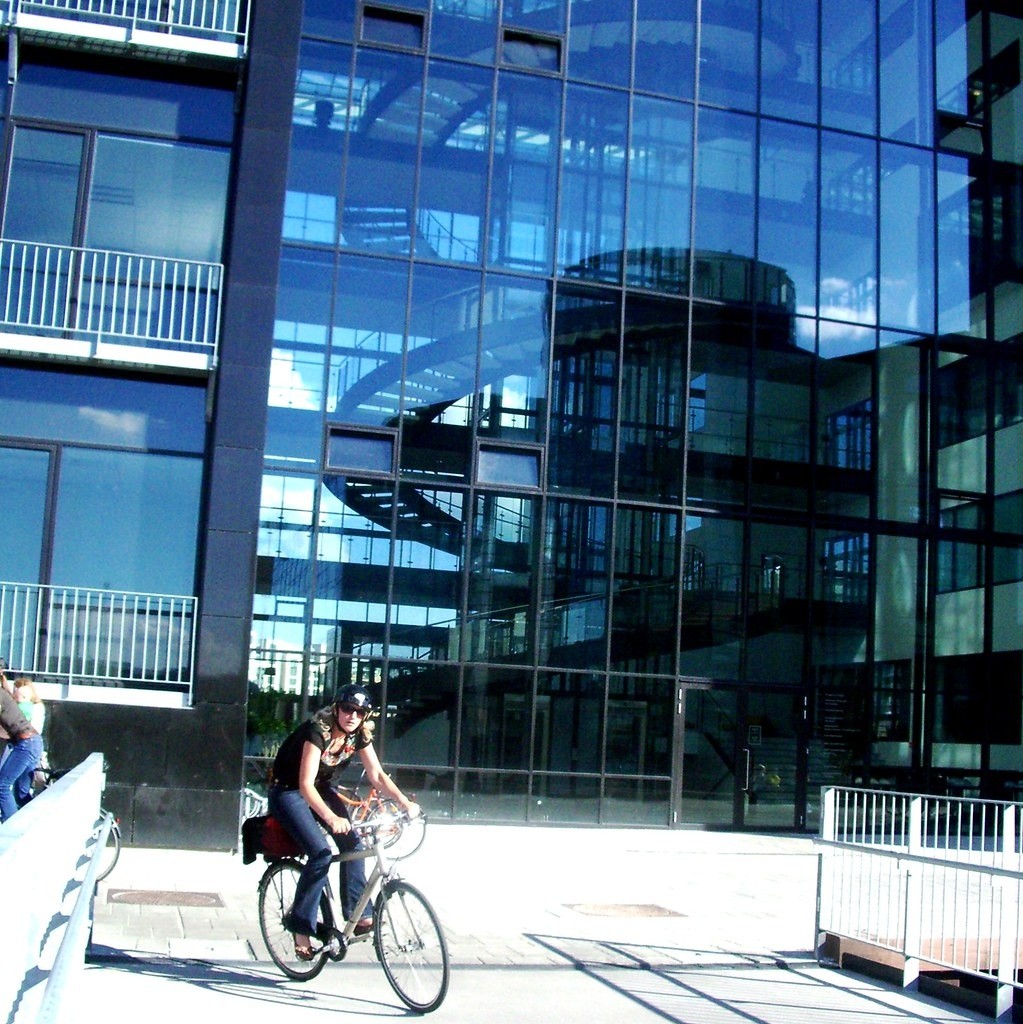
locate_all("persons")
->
[0,657,53,824]
[269,683,421,961]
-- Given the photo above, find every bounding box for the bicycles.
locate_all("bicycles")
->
[255,758,451,1015]
[20,766,122,884]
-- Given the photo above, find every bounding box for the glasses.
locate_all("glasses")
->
[339,702,368,719]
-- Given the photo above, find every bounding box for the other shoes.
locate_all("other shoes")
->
[353,921,386,936]
[293,932,313,960]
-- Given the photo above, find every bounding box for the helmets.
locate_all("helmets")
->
[332,684,373,712]
